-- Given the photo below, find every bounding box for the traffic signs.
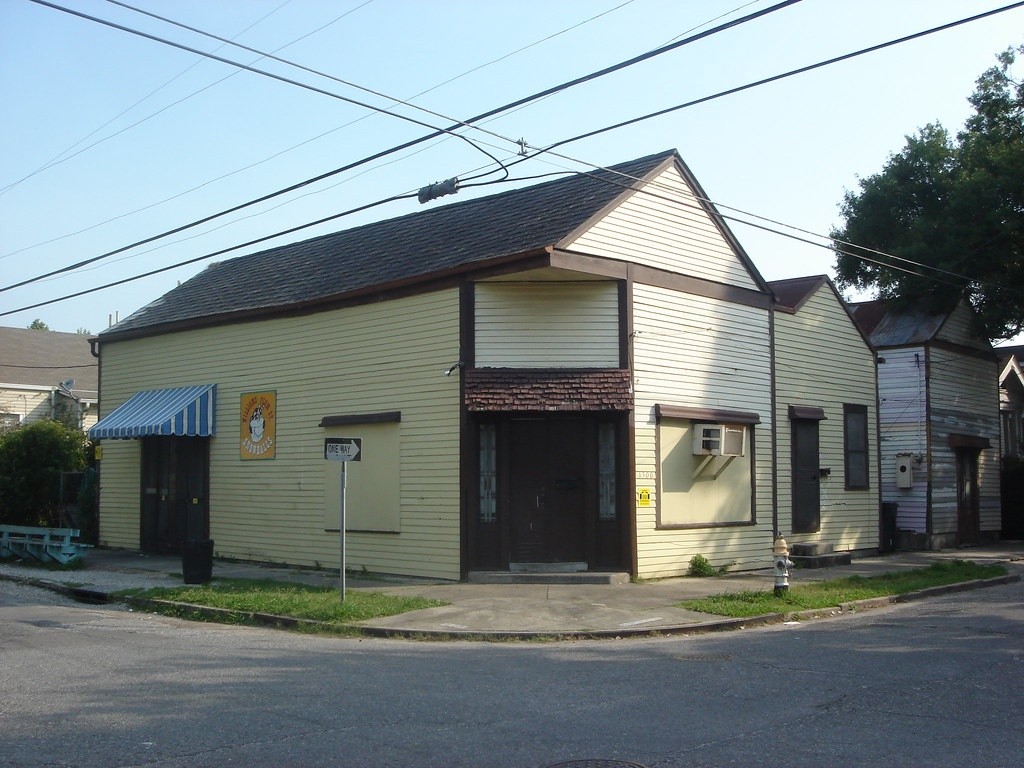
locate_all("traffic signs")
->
[323,437,362,463]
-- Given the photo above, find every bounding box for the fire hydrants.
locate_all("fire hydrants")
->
[772,532,796,596]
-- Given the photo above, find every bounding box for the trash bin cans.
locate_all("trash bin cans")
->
[183,539,213,584]
[882,501,899,551]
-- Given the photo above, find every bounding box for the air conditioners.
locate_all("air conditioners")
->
[693,424,746,456]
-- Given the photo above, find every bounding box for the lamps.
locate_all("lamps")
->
[445,360,464,376]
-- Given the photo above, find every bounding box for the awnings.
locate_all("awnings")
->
[87,383,217,440]
[950,433,994,449]
[656,403,762,424]
[788,406,828,421]
